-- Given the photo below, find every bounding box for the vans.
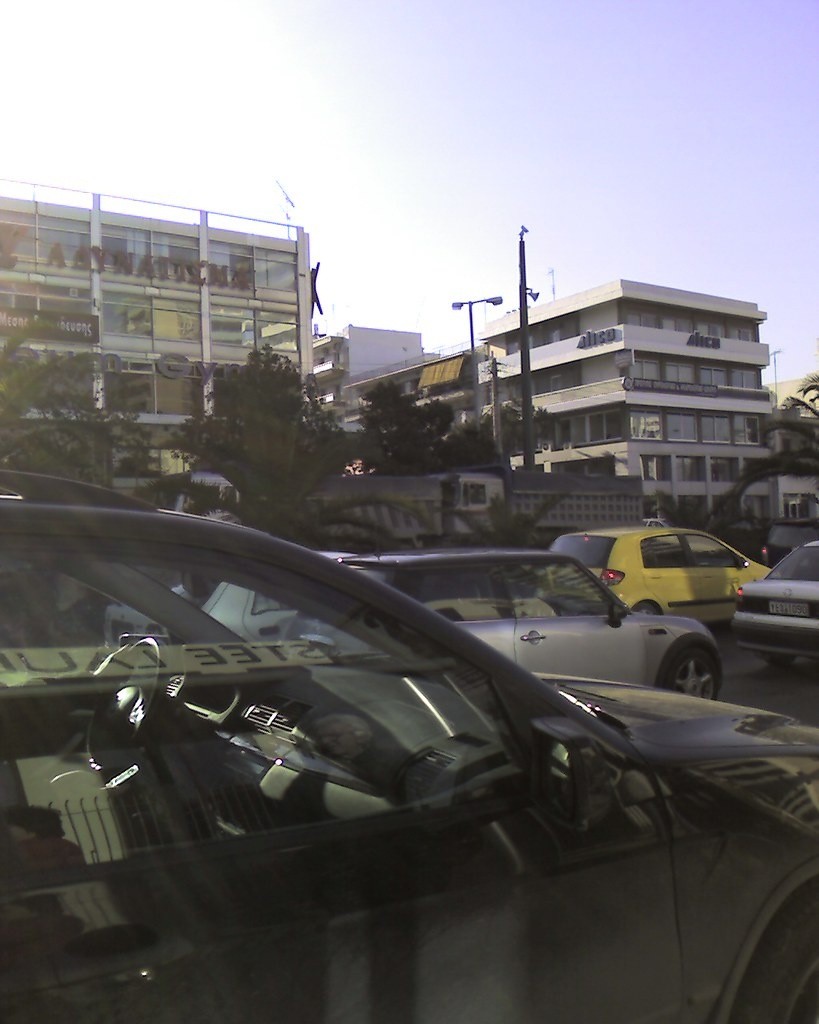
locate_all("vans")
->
[154,471,242,527]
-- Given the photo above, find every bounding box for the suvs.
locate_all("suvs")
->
[1,467,818,1024]
[761,518,819,568]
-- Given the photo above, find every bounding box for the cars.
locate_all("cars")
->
[731,540,819,667]
[535,525,776,625]
[278,547,723,705]
[104,551,362,646]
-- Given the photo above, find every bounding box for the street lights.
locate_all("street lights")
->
[451,296,503,355]
